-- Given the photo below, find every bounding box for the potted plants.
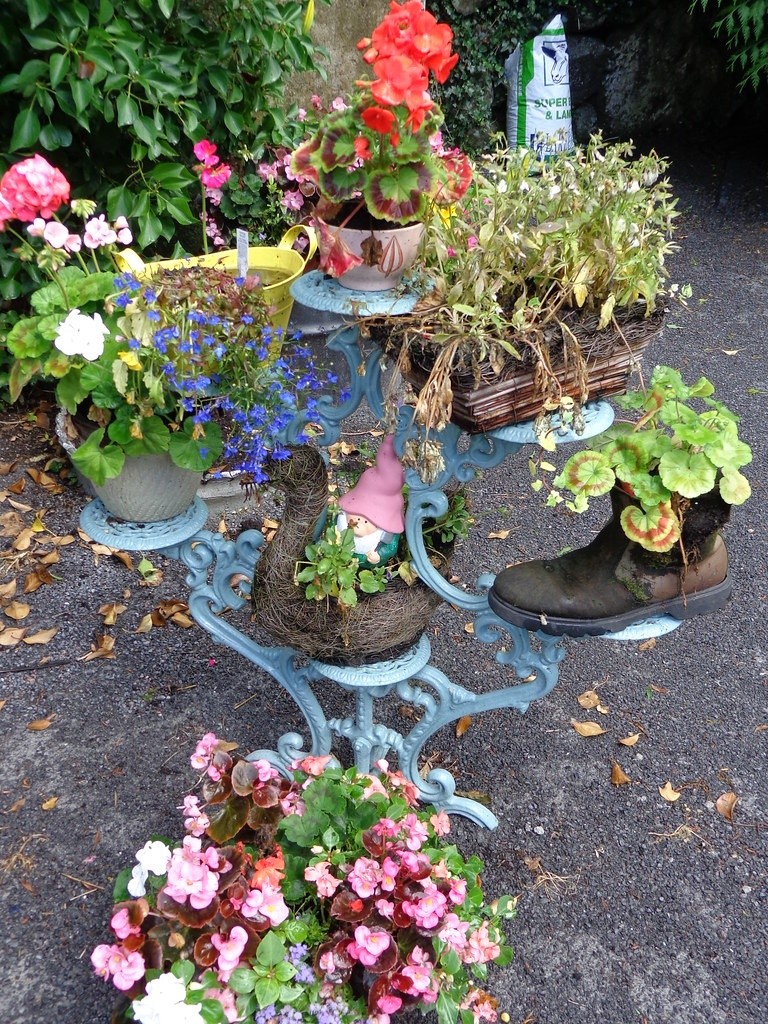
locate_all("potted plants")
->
[243,432,511,669]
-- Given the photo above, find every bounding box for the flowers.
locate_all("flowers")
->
[88,731,523,1024]
[0,0,696,493]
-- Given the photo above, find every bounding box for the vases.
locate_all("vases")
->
[54,403,202,523]
[361,291,671,433]
[312,215,425,295]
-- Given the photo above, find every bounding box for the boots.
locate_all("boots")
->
[489,456,730,638]
